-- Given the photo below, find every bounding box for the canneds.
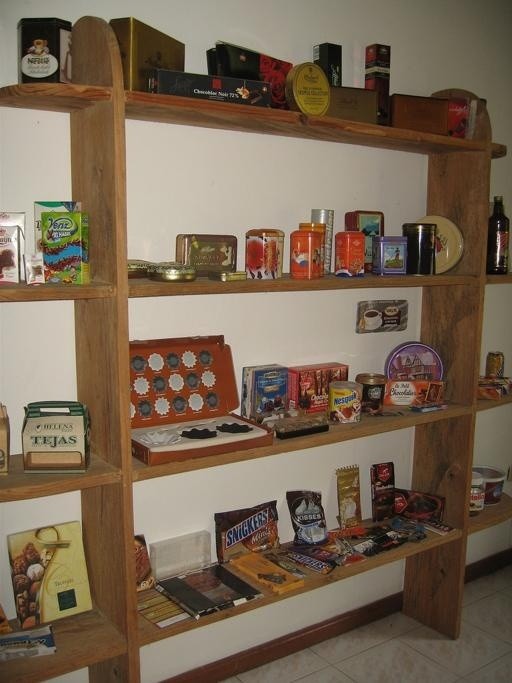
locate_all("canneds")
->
[327,381,363,424]
[486,352,505,378]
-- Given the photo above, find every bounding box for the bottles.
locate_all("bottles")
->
[487,196,510,275]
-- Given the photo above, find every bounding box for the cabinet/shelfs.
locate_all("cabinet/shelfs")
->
[0,15,512,683]
